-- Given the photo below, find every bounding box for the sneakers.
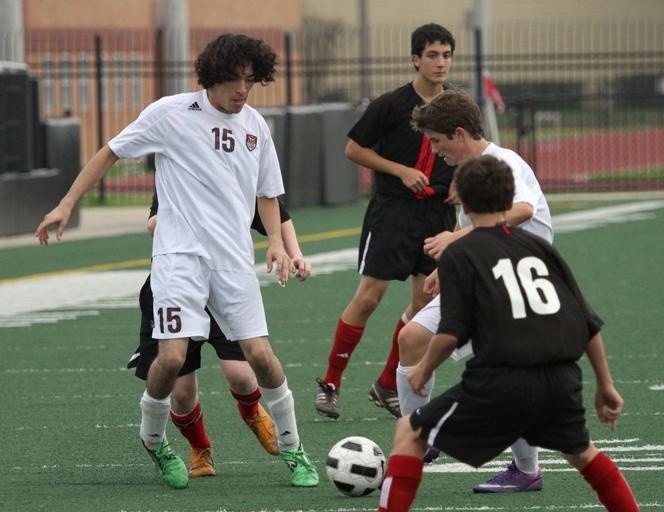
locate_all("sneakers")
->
[472,461,544,495]
[423,445,439,462]
[241,404,281,455]
[188,446,216,477]
[314,377,340,420]
[280,442,320,487]
[367,379,402,418]
[142,437,190,489]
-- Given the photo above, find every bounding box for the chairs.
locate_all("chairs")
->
[0,68,81,237]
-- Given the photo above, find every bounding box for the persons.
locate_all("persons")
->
[127,181,311,478]
[378,156,639,511]
[313,22,462,421]
[483,73,504,142]
[394,88,554,493]
[34,32,320,487]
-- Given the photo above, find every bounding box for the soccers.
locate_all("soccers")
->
[327,437,387,496]
